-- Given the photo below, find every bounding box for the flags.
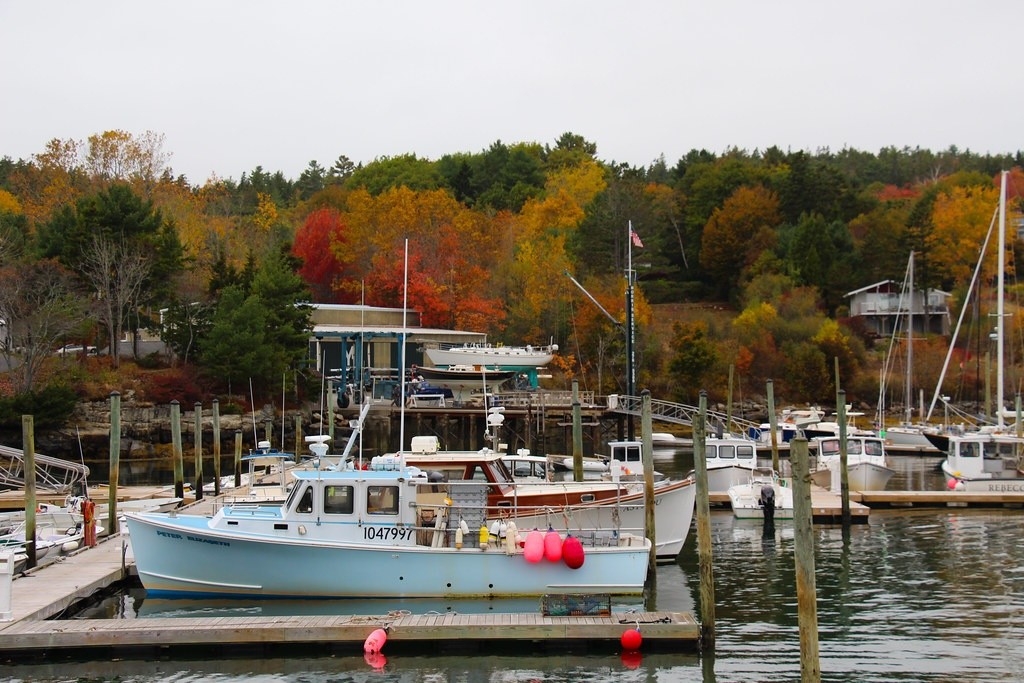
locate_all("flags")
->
[630,226,643,248]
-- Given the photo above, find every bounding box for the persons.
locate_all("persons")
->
[410,370,426,392]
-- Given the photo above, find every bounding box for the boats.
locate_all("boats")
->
[216,236,698,565]
[122,469,653,603]
[608,167,1024,525]
[0,494,105,575]
[413,335,559,390]
